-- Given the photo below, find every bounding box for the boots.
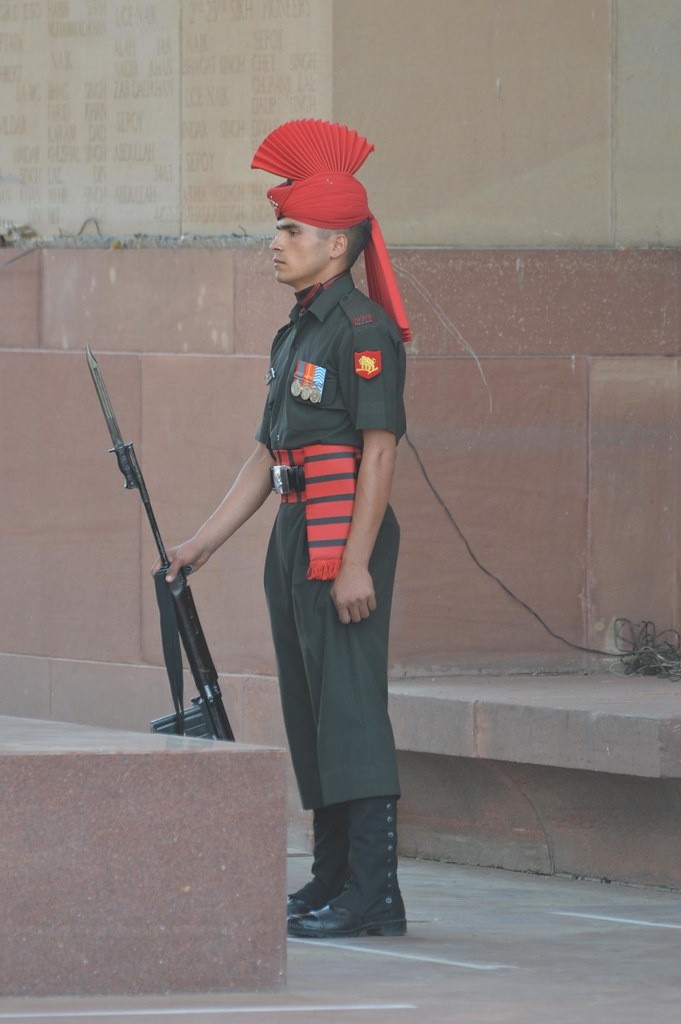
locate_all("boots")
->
[286,797,409,937]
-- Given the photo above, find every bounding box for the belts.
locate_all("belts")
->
[270,458,362,495]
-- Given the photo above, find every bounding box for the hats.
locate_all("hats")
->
[251,118,414,341]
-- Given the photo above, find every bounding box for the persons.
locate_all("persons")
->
[153,119,411,935]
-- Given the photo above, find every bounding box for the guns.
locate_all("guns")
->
[84,343,235,741]
